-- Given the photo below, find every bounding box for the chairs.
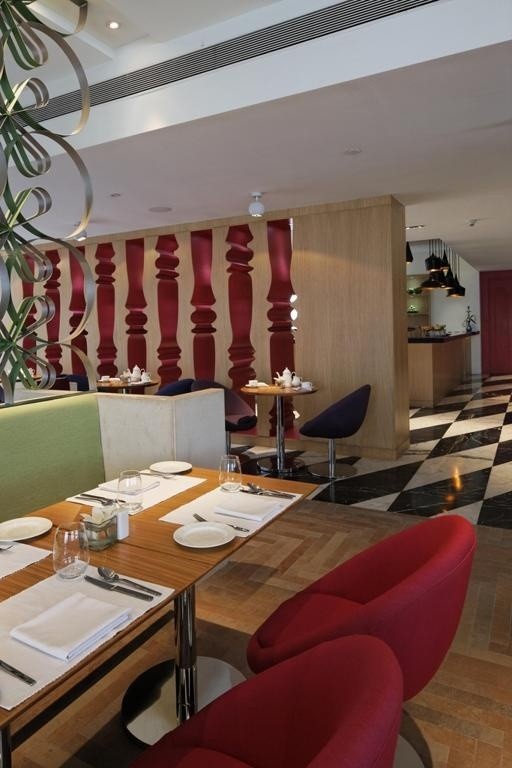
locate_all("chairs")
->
[138,632,405,768]
[187,376,257,453]
[302,382,372,480]
[153,379,193,395]
[248,515,474,767]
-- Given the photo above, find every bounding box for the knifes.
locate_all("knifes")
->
[239,488,293,500]
[83,575,153,603]
[80,493,127,505]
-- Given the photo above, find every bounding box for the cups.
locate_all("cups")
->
[218,453,243,492]
[248,378,259,386]
[101,375,110,381]
[300,380,314,390]
[115,470,145,511]
[51,520,91,580]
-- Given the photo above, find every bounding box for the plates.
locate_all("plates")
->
[245,384,260,388]
[172,521,237,550]
[98,379,113,382]
[0,515,53,544]
[148,459,193,474]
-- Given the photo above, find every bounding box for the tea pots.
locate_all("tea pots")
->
[276,367,296,388]
[127,365,146,380]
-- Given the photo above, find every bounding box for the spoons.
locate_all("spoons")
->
[75,495,113,507]
[247,482,297,499]
[97,564,163,599]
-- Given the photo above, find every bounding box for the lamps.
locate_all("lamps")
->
[247,193,264,218]
[74,222,86,241]
[422,253,464,297]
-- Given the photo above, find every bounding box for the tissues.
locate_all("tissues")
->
[78,502,119,552]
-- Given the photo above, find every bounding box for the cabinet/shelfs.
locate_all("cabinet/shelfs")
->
[407,275,430,327]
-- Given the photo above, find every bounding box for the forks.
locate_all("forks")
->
[192,512,251,533]
[140,472,175,479]
[0,544,14,552]
